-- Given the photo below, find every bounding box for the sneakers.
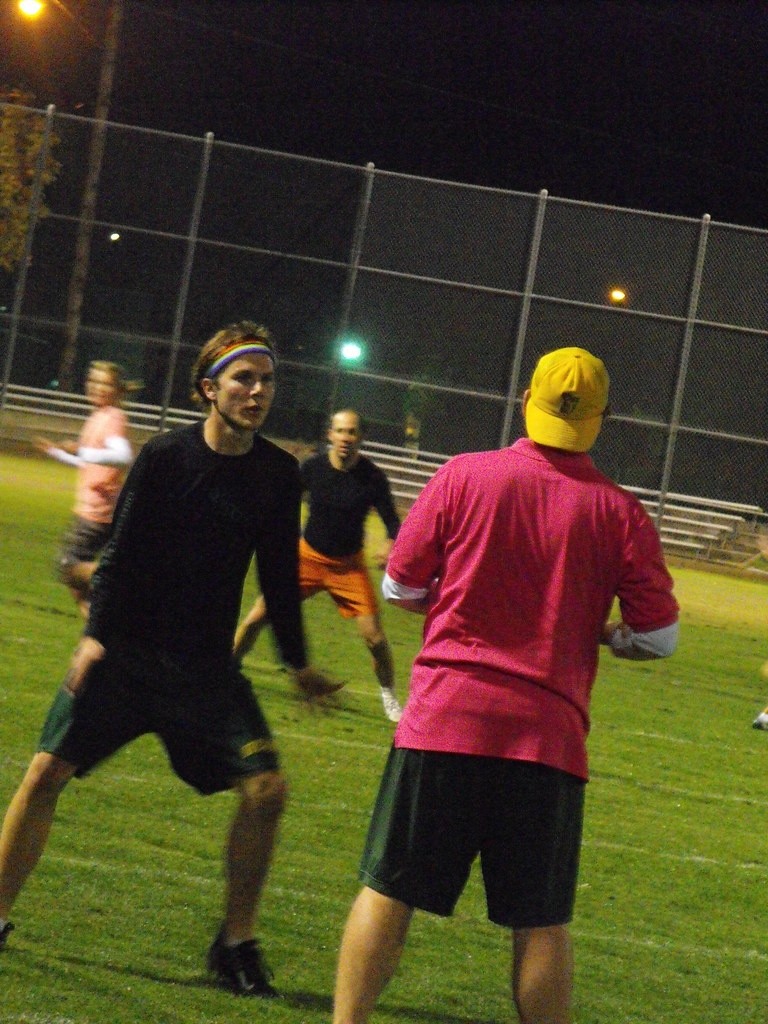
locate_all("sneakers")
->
[206,923,283,1000]
[380,686,404,722]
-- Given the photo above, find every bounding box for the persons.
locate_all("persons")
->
[331,345,679,1023]
[234,407,404,725]
[1,323,349,999]
[34,359,134,616]
[751,536,768,732]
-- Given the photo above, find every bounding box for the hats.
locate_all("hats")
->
[526,347,610,453]
